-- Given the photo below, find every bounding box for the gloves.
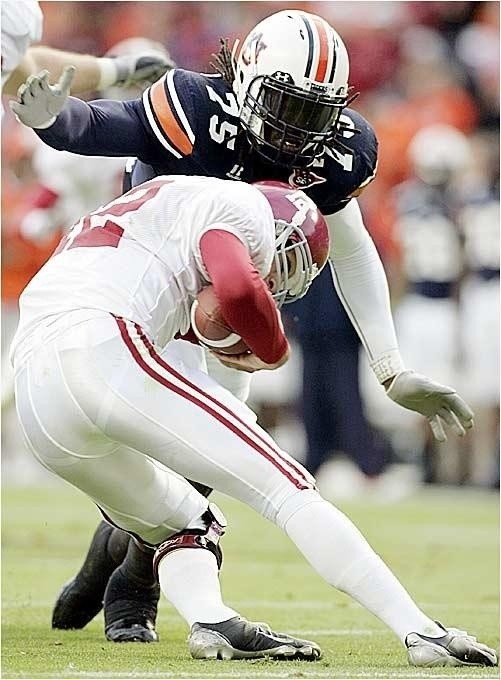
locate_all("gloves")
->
[113,53,174,93]
[383,369,473,443]
[9,63,75,128]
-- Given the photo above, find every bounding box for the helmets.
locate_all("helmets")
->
[254,179,329,312]
[230,10,357,174]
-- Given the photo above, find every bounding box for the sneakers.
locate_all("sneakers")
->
[186,615,321,661]
[51,521,160,643]
[405,620,499,669]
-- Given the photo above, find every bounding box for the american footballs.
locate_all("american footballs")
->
[190,285,249,355]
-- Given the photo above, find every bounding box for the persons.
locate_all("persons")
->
[9,174,499,669]
[9,11,471,640]
[1,1,499,486]
